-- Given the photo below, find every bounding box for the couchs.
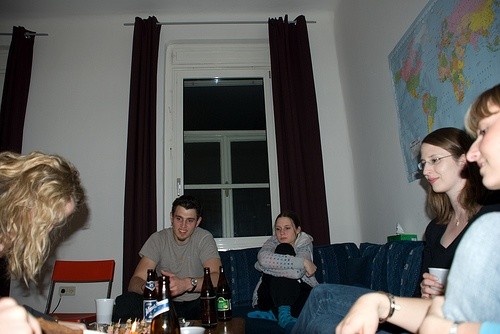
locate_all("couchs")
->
[212,242,426,334]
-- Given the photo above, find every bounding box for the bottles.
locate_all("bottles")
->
[200,268,217,329]
[215,266,233,322]
[150,274,181,334]
[142,269,157,321]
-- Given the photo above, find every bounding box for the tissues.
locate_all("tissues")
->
[387,224,417,242]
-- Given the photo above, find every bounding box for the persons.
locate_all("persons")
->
[112,195,222,324]
[336,84,500,334]
[247,211,319,329]
[0,151,88,334]
[290,128,500,334]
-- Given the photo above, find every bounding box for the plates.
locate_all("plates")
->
[180,326,206,334]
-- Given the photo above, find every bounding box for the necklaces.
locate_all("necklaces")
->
[455,213,465,226]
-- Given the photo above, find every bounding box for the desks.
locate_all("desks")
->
[185,319,288,334]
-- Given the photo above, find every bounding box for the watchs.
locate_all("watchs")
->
[187,277,197,293]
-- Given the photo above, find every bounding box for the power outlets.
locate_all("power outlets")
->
[60,286,75,296]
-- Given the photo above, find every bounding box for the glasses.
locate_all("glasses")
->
[418,154,453,170]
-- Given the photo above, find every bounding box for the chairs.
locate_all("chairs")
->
[45,260,115,323]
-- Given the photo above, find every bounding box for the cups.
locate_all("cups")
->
[428,267,449,298]
[95,298,114,325]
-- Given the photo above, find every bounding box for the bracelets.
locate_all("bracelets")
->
[377,291,395,323]
[450,321,465,334]
